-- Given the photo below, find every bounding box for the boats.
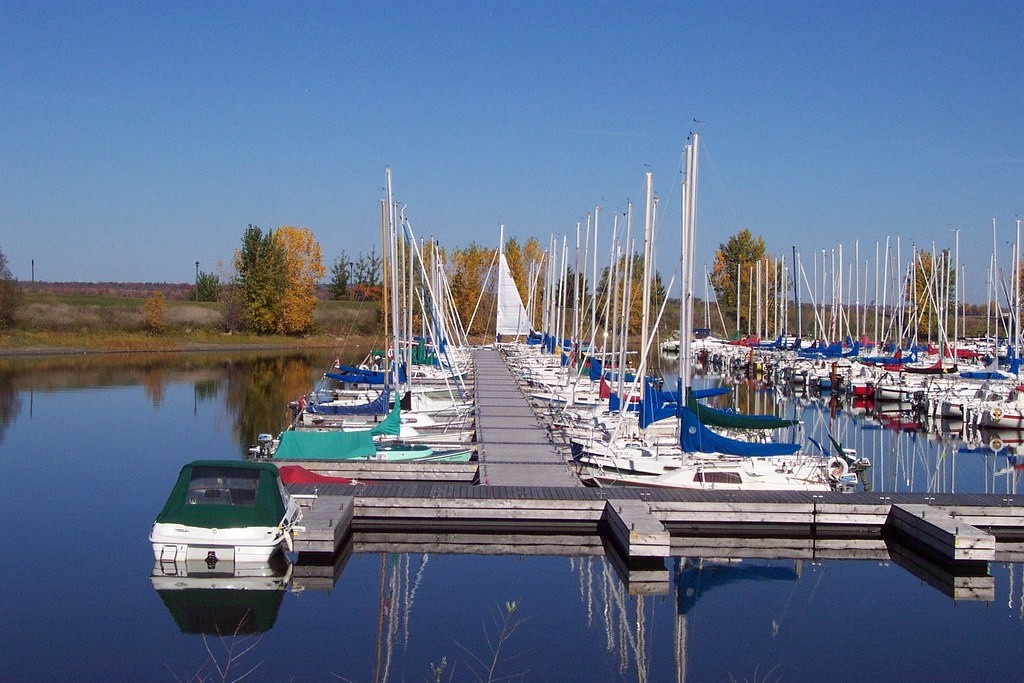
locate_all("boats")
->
[146,460,306,592]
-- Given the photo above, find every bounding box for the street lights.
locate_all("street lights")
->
[195,261,199,302]
[349,261,353,301]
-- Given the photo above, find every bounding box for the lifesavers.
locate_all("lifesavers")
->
[826,458,849,481]
[990,406,1004,419]
[989,439,1004,452]
[802,362,812,371]
[372,364,378,371]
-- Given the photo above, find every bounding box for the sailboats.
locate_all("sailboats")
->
[465,121,1023,493]
[250,167,481,462]
